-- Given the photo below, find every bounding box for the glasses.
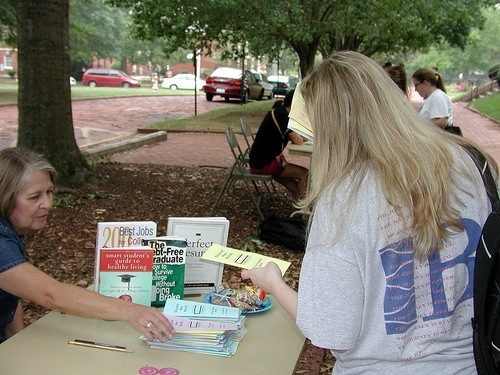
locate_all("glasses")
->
[414,80,429,88]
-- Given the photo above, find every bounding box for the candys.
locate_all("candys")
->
[207,285,272,312]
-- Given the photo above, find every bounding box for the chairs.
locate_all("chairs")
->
[212,118,279,220]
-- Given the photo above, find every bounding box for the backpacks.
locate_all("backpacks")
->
[461,144,500,375]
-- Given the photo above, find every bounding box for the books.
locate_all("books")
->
[90,216,229,319]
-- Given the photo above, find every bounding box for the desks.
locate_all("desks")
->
[280,141,311,195]
[0,286,308,375]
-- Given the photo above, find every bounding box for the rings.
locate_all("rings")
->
[146,322,153,328]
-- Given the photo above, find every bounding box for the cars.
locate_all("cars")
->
[252,72,275,100]
[203,67,265,103]
[82,68,141,88]
[161,73,206,91]
[267,75,299,96]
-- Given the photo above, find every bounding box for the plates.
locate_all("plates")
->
[203,290,273,314]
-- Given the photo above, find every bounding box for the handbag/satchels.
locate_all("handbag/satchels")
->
[260,214,307,252]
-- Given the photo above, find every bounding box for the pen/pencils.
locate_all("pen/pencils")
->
[68,339,134,353]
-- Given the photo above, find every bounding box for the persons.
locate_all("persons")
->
[240,50,500,375]
[0,147,176,346]
[248,88,311,224]
[413,67,455,129]
[383,61,406,95]
